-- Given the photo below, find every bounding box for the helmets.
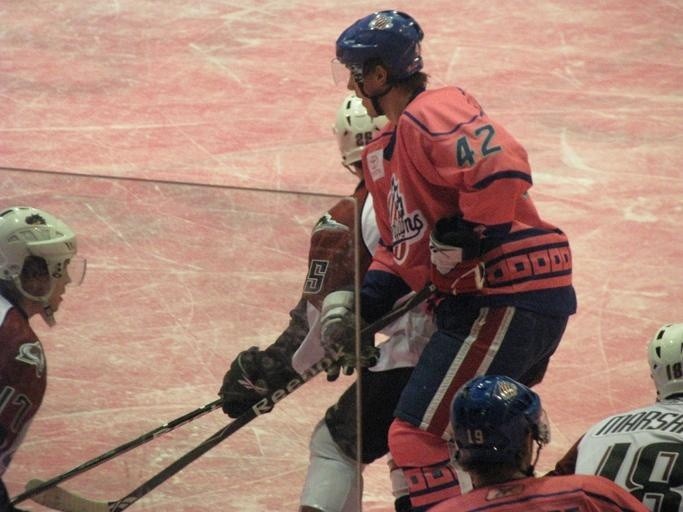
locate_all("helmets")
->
[333,10,425,84]
[331,93,389,168]
[0,206,77,282]
[646,323,683,401]
[447,373,544,466]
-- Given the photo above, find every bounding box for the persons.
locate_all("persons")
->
[219,89,441,511]
[544,321,683,511]
[0,206,78,511]
[332,7,577,512]
[426,372,653,511]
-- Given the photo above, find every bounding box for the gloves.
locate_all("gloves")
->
[426,214,487,331]
[321,312,380,384]
[216,343,268,423]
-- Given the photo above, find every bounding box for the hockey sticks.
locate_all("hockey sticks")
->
[24,284,437,512]
